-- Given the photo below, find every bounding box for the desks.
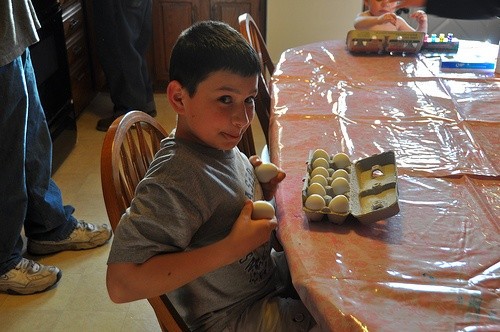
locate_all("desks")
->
[269,38,500,332]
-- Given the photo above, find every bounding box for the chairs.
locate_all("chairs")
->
[238,13,274,144]
[101,110,189,332]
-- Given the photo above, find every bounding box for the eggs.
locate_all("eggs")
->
[251,200,275,219]
[305,149,350,212]
[255,163,278,183]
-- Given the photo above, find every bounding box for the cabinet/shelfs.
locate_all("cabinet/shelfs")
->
[148,0,267,84]
[57,0,95,117]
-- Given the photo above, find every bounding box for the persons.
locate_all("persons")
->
[107,21,326,332]
[354,0,500,45]
[94,0,158,130]
[0,0,112,295]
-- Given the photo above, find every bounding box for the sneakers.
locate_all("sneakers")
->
[27,220,113,254]
[0,258,61,293]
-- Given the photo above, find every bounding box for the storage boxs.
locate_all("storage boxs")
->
[423,38,459,49]
[440,55,495,69]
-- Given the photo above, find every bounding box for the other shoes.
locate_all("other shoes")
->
[97,112,140,131]
[147,108,156,117]
